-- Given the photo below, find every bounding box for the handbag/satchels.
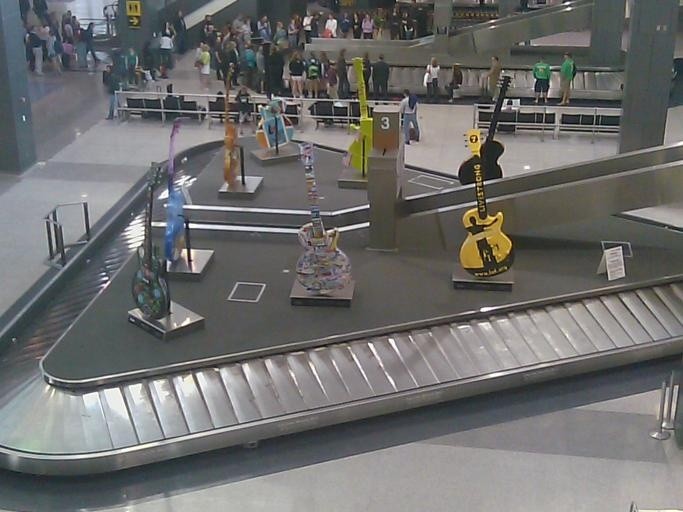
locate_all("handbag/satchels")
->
[62,40,75,57]
[53,39,65,54]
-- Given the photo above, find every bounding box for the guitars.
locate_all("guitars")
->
[164,117,189,262]
[462,128,516,276]
[132,162,170,320]
[224,62,237,184]
[296,141,351,297]
[348,56,374,173]
[458,75,515,188]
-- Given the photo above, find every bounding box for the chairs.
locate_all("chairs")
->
[473,103,622,143]
[123,96,373,130]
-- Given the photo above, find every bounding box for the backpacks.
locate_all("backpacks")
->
[306,57,320,80]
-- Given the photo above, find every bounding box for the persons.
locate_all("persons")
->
[485,55,500,104]
[532,55,551,104]
[557,50,577,106]
[480,0,483,5]
[427,57,441,98]
[424,67,432,98]
[400,88,419,144]
[520,0,528,13]
[445,64,462,103]
